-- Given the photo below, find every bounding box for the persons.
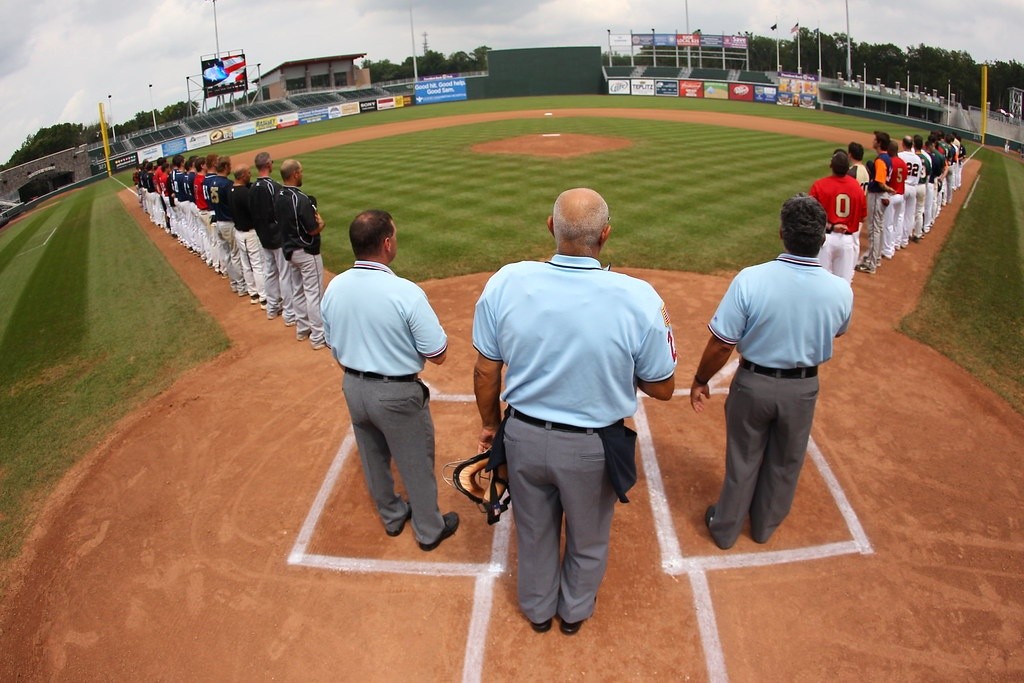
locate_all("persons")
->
[471,187,678,635]
[807,142,870,287]
[690,194,853,549]
[855,125,967,275]
[249,153,297,327]
[132,153,247,297]
[271,159,327,350]
[318,209,459,551]
[228,166,267,310]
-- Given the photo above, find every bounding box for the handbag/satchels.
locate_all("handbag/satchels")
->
[597,419,638,503]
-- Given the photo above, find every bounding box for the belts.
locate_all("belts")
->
[508,406,601,434]
[343,368,420,384]
[737,355,819,378]
[826,229,854,236]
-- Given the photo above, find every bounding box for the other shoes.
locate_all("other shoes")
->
[559,595,597,635]
[418,511,460,552]
[385,502,414,537]
[862,255,882,266]
[267,311,282,320]
[284,320,298,328]
[705,503,716,528]
[855,263,876,275]
[311,342,326,351]
[531,618,552,633]
[250,295,260,305]
[148,216,247,297]
[260,301,268,311]
[296,332,311,341]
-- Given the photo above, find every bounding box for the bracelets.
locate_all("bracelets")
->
[829,223,834,232]
[694,374,708,385]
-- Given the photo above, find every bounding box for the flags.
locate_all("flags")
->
[813,28,818,39]
[790,23,798,34]
[771,24,776,31]
